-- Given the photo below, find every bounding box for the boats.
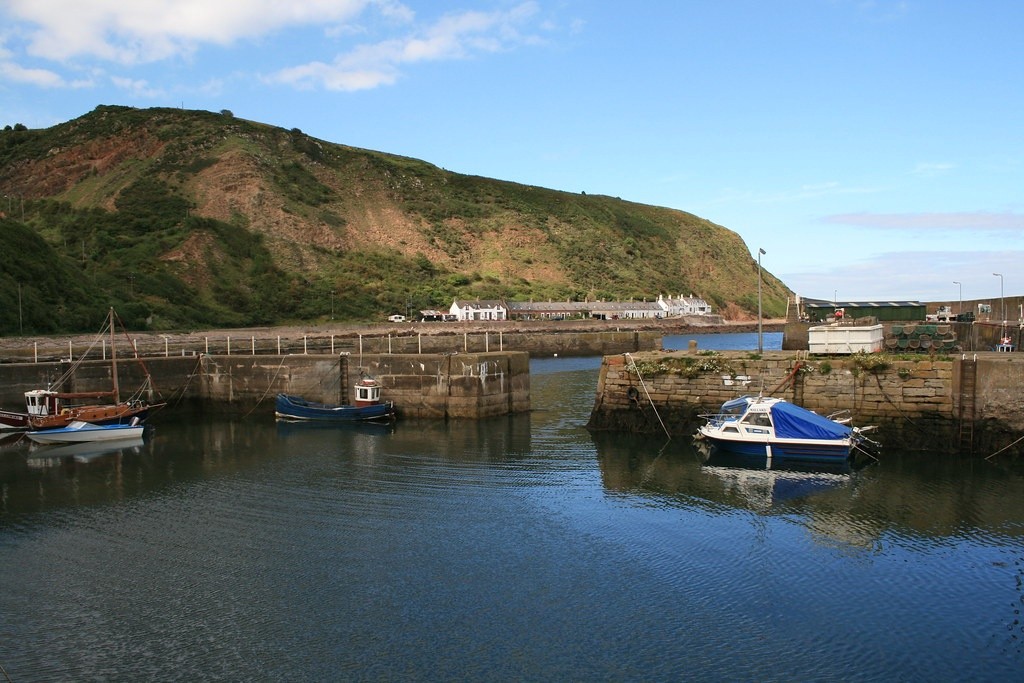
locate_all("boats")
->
[0,306,168,433]
[995,344,1015,352]
[701,449,853,501]
[274,379,394,422]
[24,416,143,444]
[699,395,860,462]
[27,438,145,464]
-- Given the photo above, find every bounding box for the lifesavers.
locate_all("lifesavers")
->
[939,305,944,311]
[61,408,71,416]
[627,387,638,400]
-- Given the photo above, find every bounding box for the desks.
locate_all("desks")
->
[996,344,1015,353]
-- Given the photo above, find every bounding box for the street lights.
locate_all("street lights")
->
[953,282,962,313]
[993,273,1003,344]
[758,247,766,354]
